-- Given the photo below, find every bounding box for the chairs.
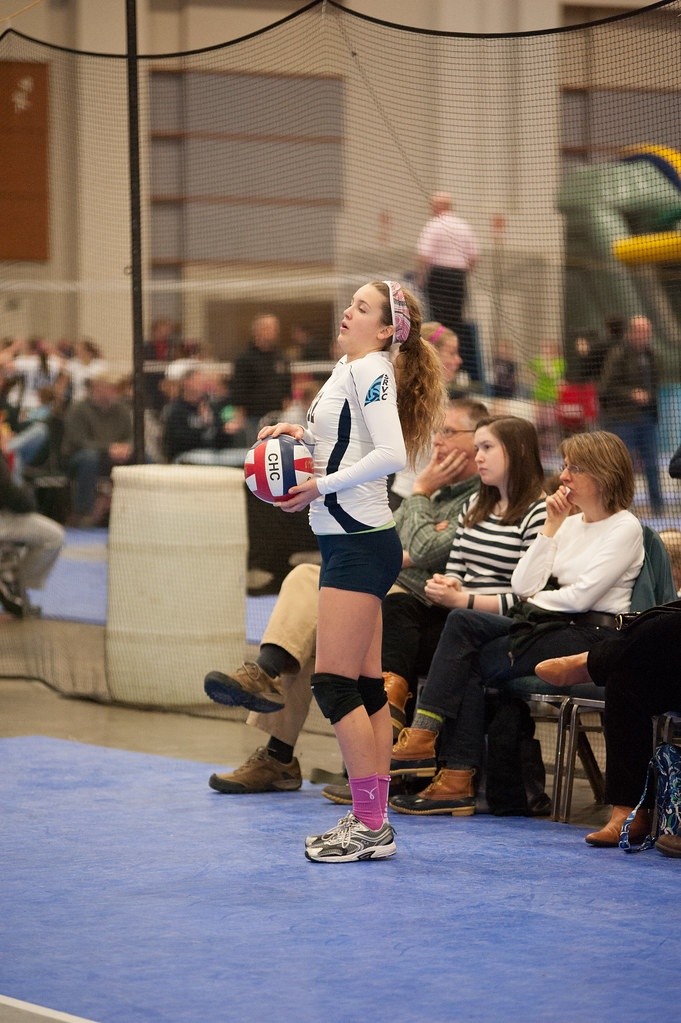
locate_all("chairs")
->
[483,524,681,841]
[0,538,37,620]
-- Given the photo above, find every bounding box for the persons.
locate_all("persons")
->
[389,431,645,815]
[204,401,549,802]
[415,192,478,353]
[420,321,461,385]
[535,445,681,856]
[257,281,441,863]
[0,316,328,617]
[490,315,667,513]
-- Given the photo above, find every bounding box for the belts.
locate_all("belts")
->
[573,612,625,631]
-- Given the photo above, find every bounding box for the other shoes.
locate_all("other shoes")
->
[388,702,406,737]
[0,570,42,617]
[322,780,405,804]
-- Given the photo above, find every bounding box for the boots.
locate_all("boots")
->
[343,727,438,781]
[389,768,477,817]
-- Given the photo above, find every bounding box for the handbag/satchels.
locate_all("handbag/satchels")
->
[619,741,681,853]
[484,698,553,817]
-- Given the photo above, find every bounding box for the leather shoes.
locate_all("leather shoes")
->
[585,805,650,845]
[534,651,593,687]
[655,835,681,858]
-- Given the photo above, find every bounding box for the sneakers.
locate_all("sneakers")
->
[304,810,397,862]
[209,745,302,792]
[204,661,286,714]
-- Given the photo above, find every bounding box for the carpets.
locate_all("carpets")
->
[0,736,681,1023]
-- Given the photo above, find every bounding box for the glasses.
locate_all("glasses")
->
[559,464,584,474]
[432,426,476,439]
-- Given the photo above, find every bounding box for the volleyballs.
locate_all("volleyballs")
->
[243,433,315,505]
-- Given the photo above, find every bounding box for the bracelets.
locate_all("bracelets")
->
[467,594,474,609]
